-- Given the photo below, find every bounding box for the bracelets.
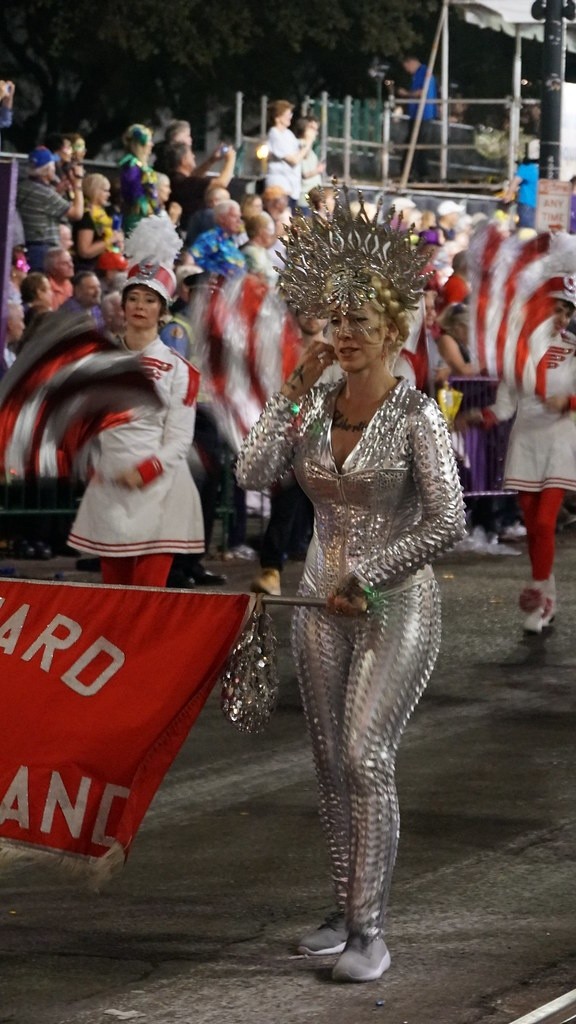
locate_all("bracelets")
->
[75,186,82,192]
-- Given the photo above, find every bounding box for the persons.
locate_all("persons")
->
[234,177,467,978]
[467,230,576,633]
[396,52,436,186]
[0,79,576,554]
[66,264,206,587]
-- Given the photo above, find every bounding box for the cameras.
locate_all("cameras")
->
[61,162,77,176]
[220,146,228,156]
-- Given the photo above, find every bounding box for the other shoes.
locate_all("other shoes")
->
[167,574,194,588]
[193,568,227,585]
[501,520,528,539]
[224,544,258,562]
[556,507,576,530]
[487,543,522,556]
[486,532,500,544]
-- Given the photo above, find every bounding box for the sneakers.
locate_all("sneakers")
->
[250,568,281,596]
[297,912,350,955]
[331,932,391,982]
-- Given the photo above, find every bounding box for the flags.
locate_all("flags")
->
[0,580,253,880]
[0,313,165,483]
[464,223,554,404]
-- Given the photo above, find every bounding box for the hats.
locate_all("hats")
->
[537,227,576,308]
[121,209,184,309]
[29,145,60,168]
[413,231,443,247]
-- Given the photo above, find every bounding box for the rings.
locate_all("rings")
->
[319,357,324,363]
[335,609,343,617]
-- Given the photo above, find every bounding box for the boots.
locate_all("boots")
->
[519,573,557,633]
